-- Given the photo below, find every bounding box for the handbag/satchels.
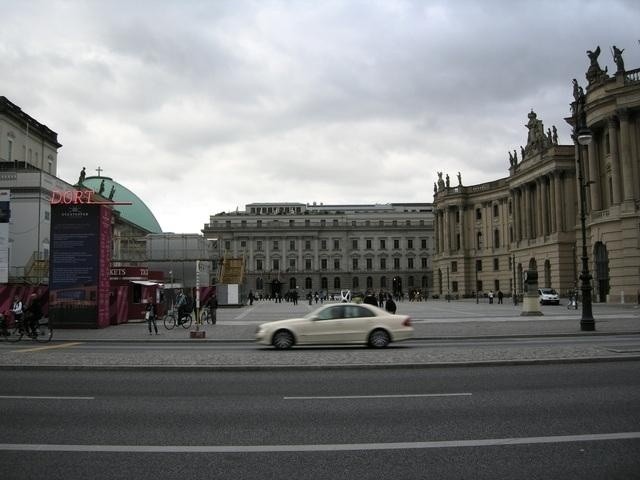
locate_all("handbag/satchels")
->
[145,311,150,321]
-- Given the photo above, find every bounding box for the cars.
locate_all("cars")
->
[255,302,415,350]
[538,287,560,305]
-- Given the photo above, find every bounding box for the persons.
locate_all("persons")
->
[612,45,626,71]
[248,287,431,307]
[174,289,186,327]
[578,86,583,97]
[572,290,579,310]
[573,77,579,99]
[192,294,201,325]
[25,293,43,341]
[109,185,116,199]
[99,179,105,194]
[385,295,397,314]
[9,294,23,336]
[497,290,504,304]
[566,291,573,312]
[508,108,558,168]
[204,292,218,325]
[433,172,463,194]
[141,296,161,335]
[488,290,494,304]
[77,166,86,185]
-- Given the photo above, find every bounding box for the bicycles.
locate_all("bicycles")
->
[4,314,52,343]
[164,305,212,330]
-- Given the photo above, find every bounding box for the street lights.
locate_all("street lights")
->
[573,127,596,331]
